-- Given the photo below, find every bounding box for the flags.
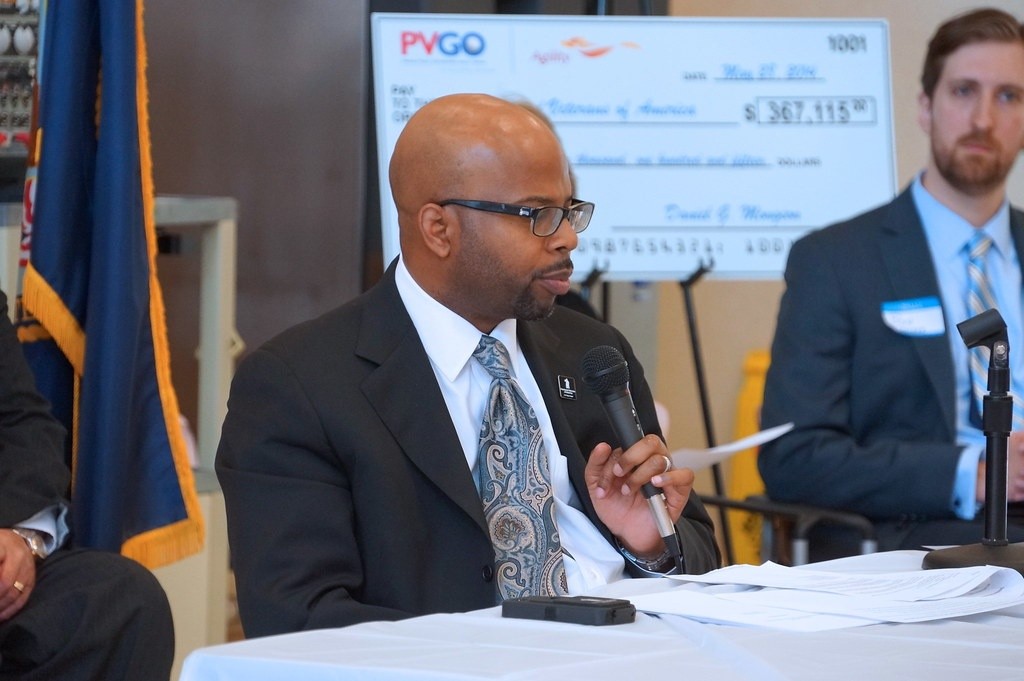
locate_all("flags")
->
[11,0,203,566]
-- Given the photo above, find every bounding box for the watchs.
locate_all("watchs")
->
[11,528,48,559]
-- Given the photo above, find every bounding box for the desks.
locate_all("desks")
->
[181,545,1024,681]
[0,196,243,472]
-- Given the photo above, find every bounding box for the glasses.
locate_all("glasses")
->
[439,195,594,238]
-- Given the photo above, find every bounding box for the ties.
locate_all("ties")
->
[473,334,567,604]
[964,228,1007,421]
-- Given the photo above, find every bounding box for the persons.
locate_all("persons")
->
[0,291,175,681]
[757,7,1024,564]
[215,95,720,640]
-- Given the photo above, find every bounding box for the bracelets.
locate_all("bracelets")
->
[620,546,670,571]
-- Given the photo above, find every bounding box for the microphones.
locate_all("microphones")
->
[583,346,681,557]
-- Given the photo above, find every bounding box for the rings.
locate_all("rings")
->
[663,455,670,473]
[14,580,24,592]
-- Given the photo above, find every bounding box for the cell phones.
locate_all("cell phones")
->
[502,596,635,626]
